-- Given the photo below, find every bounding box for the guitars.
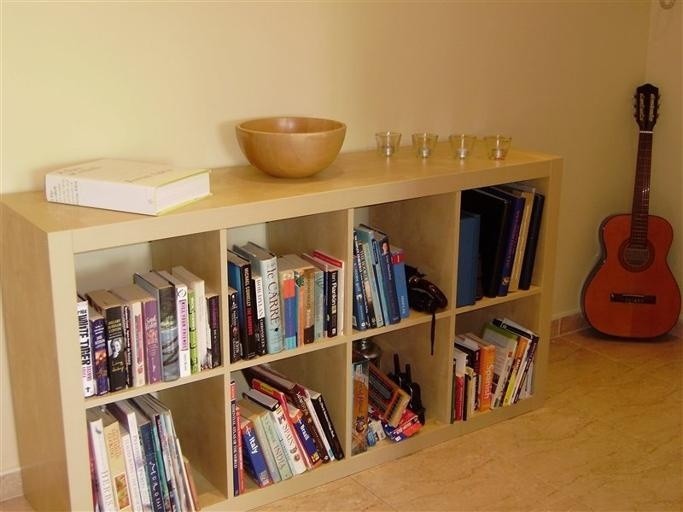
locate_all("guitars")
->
[580,84,681,338]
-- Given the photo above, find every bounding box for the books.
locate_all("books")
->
[42,157,214,217]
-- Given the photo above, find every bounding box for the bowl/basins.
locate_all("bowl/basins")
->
[234,116,346,179]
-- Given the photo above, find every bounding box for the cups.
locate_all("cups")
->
[448,134,477,160]
[374,131,402,158]
[411,132,439,160]
[483,135,512,162]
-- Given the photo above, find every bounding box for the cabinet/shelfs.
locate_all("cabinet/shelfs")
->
[1,138,565,512]
